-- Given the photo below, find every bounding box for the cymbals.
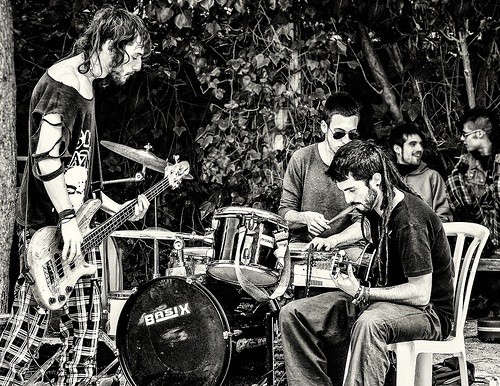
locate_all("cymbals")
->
[99,138,195,179]
[110,226,195,241]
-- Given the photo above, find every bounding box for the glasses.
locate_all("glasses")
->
[460,130,485,138]
[325,120,360,140]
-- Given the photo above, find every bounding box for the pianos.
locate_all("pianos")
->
[165,245,370,291]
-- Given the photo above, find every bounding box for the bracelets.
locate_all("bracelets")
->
[355,286,363,298]
[56,209,77,222]
[364,286,374,306]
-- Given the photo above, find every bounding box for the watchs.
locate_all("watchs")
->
[351,286,366,305]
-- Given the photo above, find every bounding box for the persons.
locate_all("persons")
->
[0,6,151,386]
[278,91,500,386]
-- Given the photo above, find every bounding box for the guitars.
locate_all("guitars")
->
[28,156,190,312]
[329,241,378,287]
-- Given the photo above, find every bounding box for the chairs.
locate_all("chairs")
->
[374,221,490,386]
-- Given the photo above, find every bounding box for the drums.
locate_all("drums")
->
[105,287,141,338]
[208,204,289,287]
[114,276,272,386]
